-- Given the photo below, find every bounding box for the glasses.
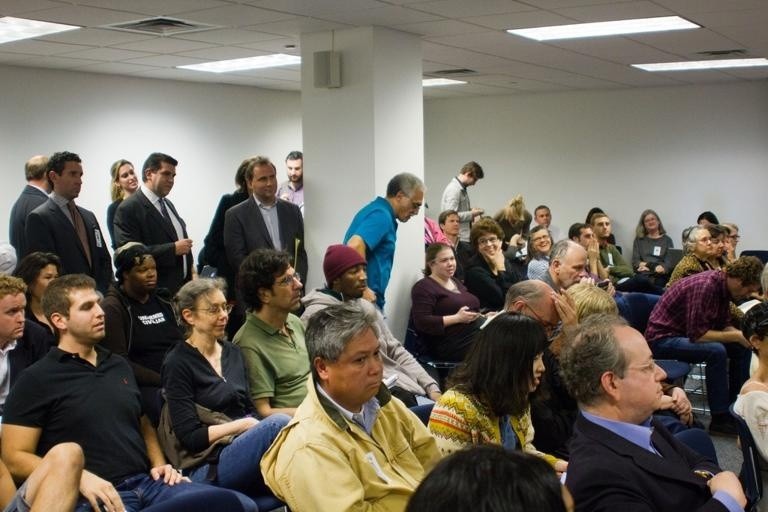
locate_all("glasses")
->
[476,236,499,245]
[271,273,300,286]
[627,360,655,371]
[513,299,563,331]
[409,196,420,210]
[197,305,233,314]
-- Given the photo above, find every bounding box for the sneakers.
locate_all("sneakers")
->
[710,416,735,436]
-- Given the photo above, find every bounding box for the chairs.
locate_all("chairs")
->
[728,401,768,512]
[616,292,706,416]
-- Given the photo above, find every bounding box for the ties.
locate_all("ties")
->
[160,199,178,240]
[68,202,91,266]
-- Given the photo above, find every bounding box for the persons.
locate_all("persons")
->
[0,149,768,512]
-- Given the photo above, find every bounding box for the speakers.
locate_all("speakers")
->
[312,51,340,88]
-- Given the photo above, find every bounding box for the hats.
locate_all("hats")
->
[323,244,367,282]
